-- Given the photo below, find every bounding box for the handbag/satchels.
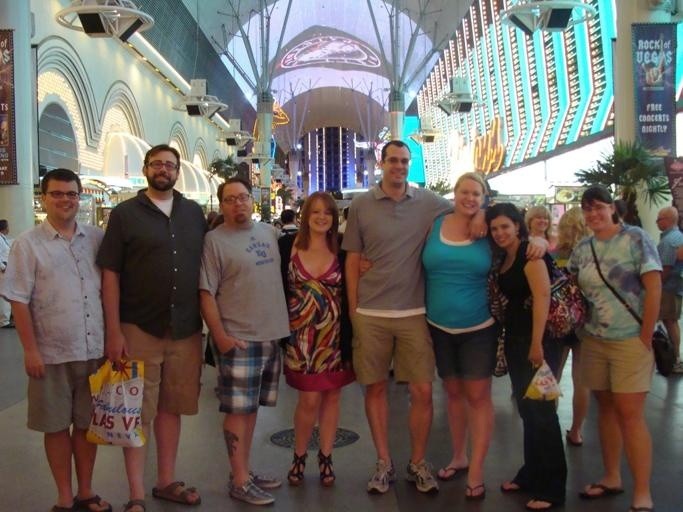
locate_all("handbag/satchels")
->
[492,327,510,377]
[589,239,676,379]
[86,358,146,449]
[522,244,589,341]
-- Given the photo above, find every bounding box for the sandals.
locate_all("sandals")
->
[288,451,306,483]
[318,449,335,486]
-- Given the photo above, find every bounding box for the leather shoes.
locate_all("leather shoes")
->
[1,324,12,328]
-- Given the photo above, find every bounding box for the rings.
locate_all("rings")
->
[480,231,484,234]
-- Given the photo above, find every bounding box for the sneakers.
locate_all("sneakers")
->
[228,478,275,506]
[367,460,395,495]
[230,468,285,489]
[674,363,683,375]
[405,462,440,495]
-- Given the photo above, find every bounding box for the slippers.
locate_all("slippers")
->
[153,479,202,507]
[577,479,626,498]
[500,478,528,489]
[566,426,582,445]
[467,482,487,501]
[630,499,652,512]
[123,497,141,508]
[53,501,81,511]
[75,493,113,512]
[438,460,469,480]
[526,497,556,508]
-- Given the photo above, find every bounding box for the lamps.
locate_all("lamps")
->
[54,0,153,44]
[172,79,227,119]
[216,120,255,148]
[408,118,440,146]
[236,150,247,157]
[500,0,596,35]
[431,77,479,116]
[244,143,271,166]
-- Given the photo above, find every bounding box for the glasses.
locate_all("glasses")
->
[145,160,180,172]
[382,157,411,165]
[44,189,81,201]
[223,193,255,203]
[579,204,612,216]
[655,217,672,223]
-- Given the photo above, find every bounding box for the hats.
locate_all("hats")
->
[582,184,622,225]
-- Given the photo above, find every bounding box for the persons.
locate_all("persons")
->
[95,144,208,511]
[207,211,224,231]
[487,203,592,510]
[1,220,15,328]
[564,186,663,511]
[614,199,627,224]
[338,207,349,233]
[0,169,113,512]
[341,140,489,495]
[549,207,594,446]
[357,172,550,500]
[278,192,348,488]
[524,205,554,240]
[259,209,301,235]
[199,177,291,505]
[655,206,683,374]
